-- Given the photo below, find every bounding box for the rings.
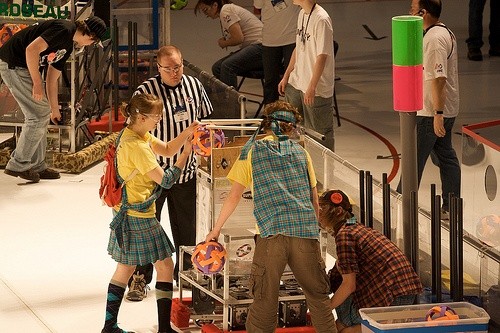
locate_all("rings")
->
[438,129,441,131]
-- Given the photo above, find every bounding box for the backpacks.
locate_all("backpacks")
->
[100,127,139,208]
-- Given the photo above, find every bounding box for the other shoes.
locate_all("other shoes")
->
[40,168,61,179]
[4,168,40,182]
[439,207,450,220]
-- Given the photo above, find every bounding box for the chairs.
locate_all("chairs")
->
[333,40,341,125]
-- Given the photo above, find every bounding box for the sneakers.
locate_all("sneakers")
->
[126,273,150,301]
[176,268,210,288]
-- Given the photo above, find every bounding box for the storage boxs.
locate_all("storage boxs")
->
[359,302,490,333]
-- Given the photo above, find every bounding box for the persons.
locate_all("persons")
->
[205,102,337,333]
[126,45,215,300]
[195,0,264,90]
[278,0,335,153]
[465,0,500,61]
[396,0,461,219]
[100,94,203,333]
[253,0,301,108]
[0,16,107,182]
[319,189,423,326]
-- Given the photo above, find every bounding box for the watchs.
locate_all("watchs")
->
[434,110,443,115]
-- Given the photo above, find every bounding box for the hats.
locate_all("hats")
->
[318,189,351,212]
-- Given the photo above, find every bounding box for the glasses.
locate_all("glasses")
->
[157,58,183,73]
[138,112,163,122]
